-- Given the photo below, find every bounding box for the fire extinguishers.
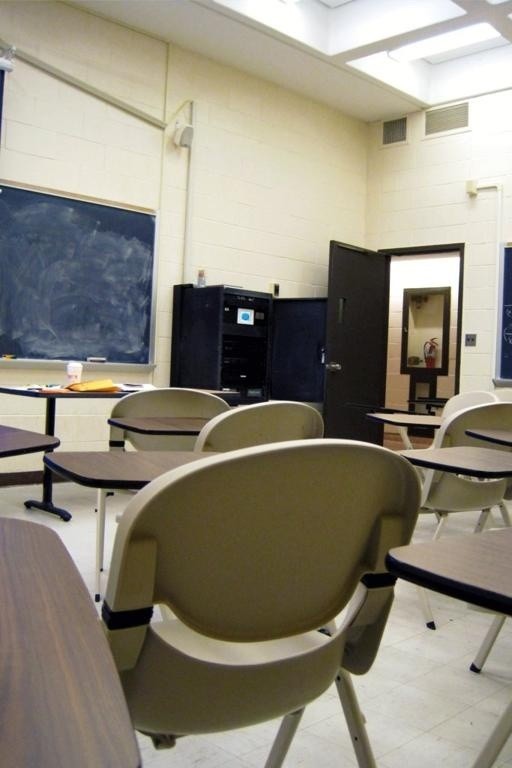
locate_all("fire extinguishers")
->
[423,338,439,368]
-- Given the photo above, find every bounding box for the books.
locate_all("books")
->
[115,381,158,391]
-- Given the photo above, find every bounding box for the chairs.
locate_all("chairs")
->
[0,381,510,767]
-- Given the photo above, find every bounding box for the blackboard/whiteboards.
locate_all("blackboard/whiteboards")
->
[0,179,158,372]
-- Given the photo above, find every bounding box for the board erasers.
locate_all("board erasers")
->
[87,356,107,362]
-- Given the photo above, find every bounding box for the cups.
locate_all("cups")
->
[66,362,84,387]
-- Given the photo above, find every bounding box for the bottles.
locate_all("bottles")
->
[196,269,205,287]
